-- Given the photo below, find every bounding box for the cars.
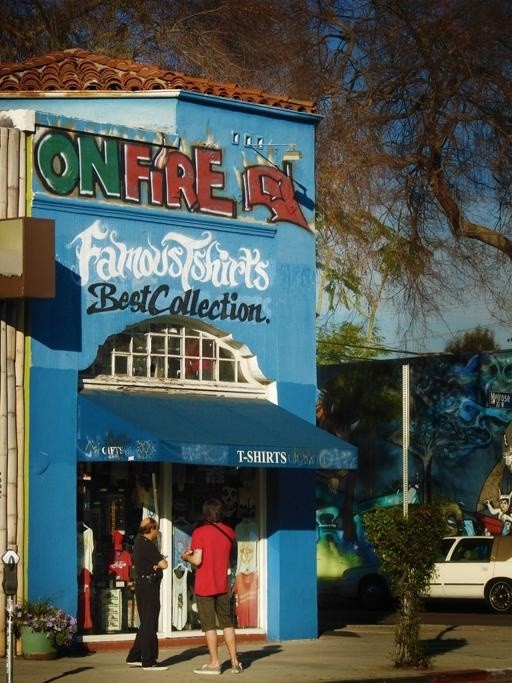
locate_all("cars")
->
[341,536,512,614]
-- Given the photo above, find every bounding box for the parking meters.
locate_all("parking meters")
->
[1,548,20,683]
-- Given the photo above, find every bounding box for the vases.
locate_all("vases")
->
[19,623,60,660]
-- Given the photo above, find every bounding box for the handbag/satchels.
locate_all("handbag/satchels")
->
[230,540,239,567]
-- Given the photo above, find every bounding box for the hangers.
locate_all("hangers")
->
[171,515,192,526]
[238,517,253,523]
[76,521,89,531]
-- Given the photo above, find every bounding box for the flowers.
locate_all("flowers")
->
[11,596,79,650]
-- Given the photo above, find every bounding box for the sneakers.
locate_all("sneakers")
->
[140,660,172,672]
[125,660,142,666]
[191,662,224,676]
[231,660,244,675]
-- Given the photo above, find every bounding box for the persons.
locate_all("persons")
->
[485,488,512,521]
[182,496,245,674]
[124,517,170,671]
